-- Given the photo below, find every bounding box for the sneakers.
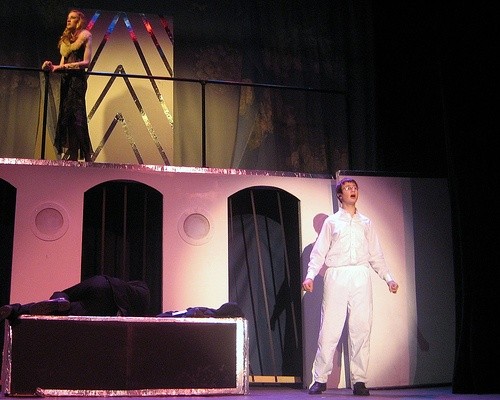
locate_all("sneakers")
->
[353,382,369,395]
[309,382,326,394]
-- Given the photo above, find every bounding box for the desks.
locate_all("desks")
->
[1,315,249,397]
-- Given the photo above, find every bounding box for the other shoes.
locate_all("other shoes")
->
[0,303,21,321]
[85,158,94,163]
[29,299,71,315]
[67,157,78,162]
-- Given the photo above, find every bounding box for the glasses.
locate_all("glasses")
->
[341,186,358,191]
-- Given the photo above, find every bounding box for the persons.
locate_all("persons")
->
[0,274,152,319]
[301,177,398,396]
[42,9,96,162]
